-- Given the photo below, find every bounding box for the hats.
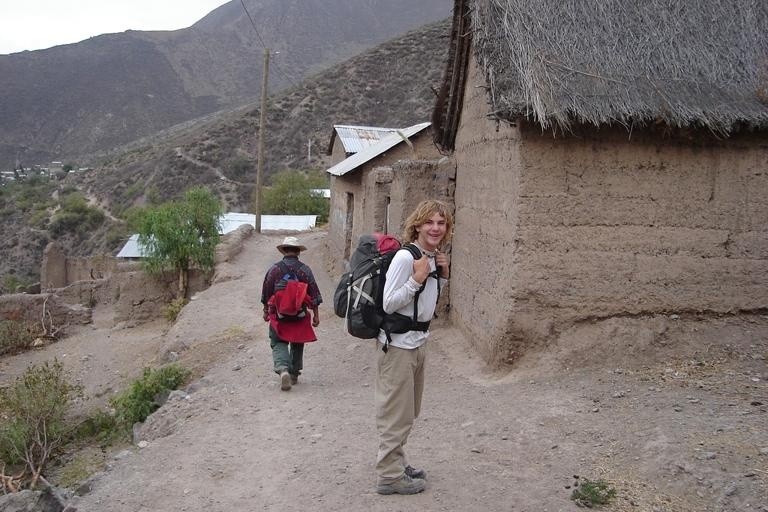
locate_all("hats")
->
[276,237,307,256]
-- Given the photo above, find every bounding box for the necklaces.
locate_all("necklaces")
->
[416,240,437,259]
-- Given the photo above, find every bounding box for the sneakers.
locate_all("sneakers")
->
[377,464,426,496]
[281,372,291,391]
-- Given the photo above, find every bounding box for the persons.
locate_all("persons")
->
[260,237,323,391]
[375,200,452,495]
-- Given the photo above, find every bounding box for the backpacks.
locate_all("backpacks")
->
[334,233,426,338]
[275,260,307,322]
